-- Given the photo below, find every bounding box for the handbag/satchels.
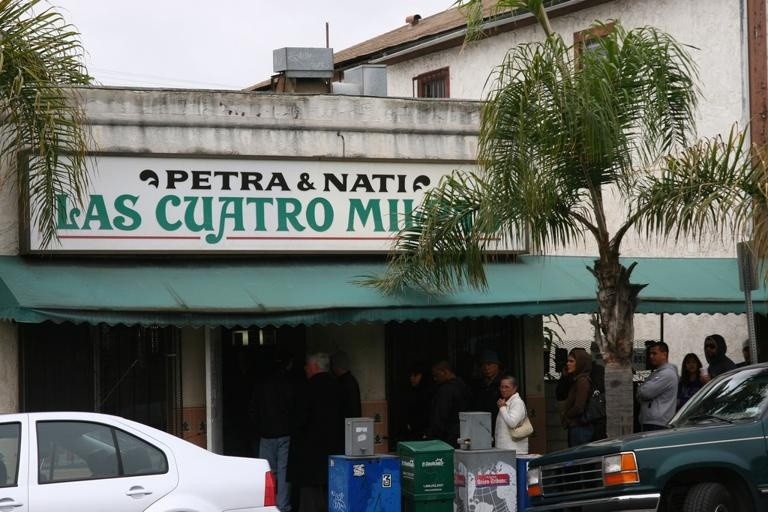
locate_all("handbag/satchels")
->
[508,417,534,442]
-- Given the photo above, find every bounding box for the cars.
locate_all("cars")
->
[1,410,281,512]
[526,361,768,512]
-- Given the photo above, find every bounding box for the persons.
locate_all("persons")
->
[253,345,363,512]
[403,347,501,445]
[634,332,757,430]
[495,375,533,455]
[556,346,605,448]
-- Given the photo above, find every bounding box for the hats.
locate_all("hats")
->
[475,348,498,364]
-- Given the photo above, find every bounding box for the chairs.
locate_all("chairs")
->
[85,447,154,477]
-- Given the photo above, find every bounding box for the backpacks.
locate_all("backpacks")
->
[583,387,606,424]
